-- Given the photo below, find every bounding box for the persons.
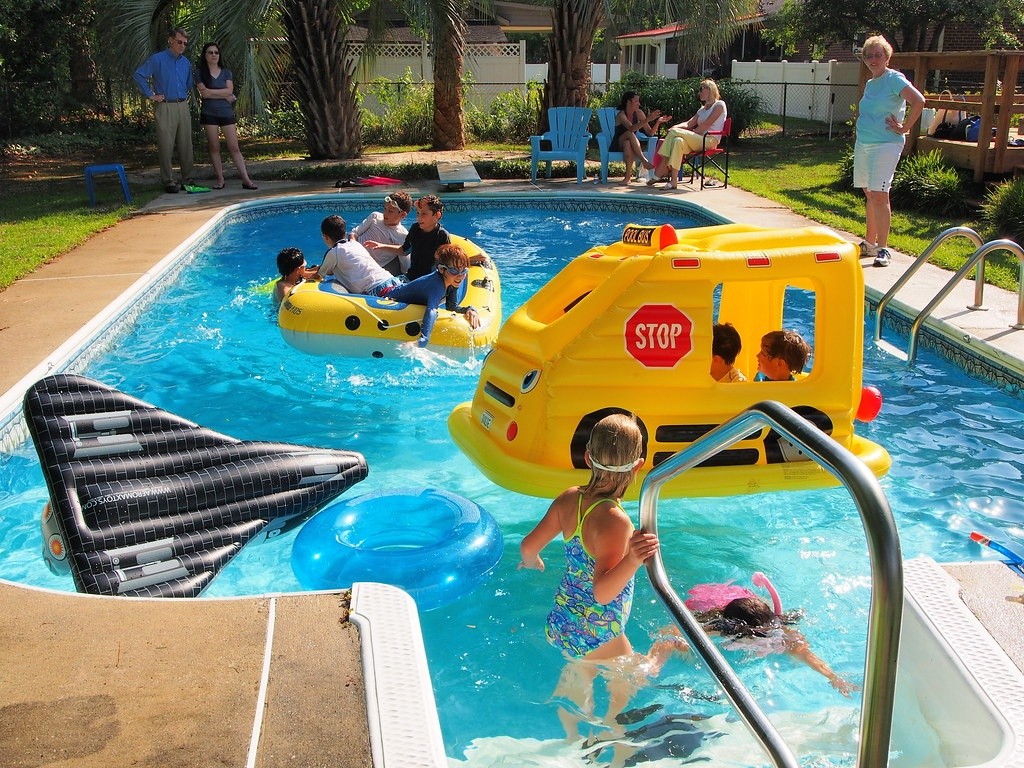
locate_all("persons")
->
[194,42,258,190]
[273,247,317,304]
[753,331,812,382]
[710,322,747,382]
[609,91,672,186]
[380,244,481,348]
[348,191,411,275]
[853,35,925,267]
[646,80,727,191]
[306,215,402,297]
[132,27,204,193]
[518,414,659,656]
[363,195,451,284]
[722,597,774,628]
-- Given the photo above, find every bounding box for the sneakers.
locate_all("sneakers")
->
[873,248,891,267]
[859,240,878,256]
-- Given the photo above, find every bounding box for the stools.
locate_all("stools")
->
[81,162,131,206]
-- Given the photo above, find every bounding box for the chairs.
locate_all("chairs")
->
[654,115,735,190]
[527,104,594,187]
[596,105,656,189]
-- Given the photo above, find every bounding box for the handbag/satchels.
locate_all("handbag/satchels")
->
[921,90,981,142]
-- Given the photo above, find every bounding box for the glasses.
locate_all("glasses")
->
[699,87,708,92]
[172,38,187,46]
[865,52,885,59]
[206,51,219,54]
[438,264,468,275]
[384,195,406,220]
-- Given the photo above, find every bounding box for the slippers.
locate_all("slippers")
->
[659,183,677,190]
[647,175,669,185]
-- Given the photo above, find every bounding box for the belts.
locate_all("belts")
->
[155,98,186,103]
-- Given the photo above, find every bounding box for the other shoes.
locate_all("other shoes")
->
[643,162,654,169]
[705,174,718,186]
[212,182,226,190]
[181,182,203,191]
[165,184,179,193]
[1008,135,1024,146]
[242,182,258,189]
[618,178,631,185]
